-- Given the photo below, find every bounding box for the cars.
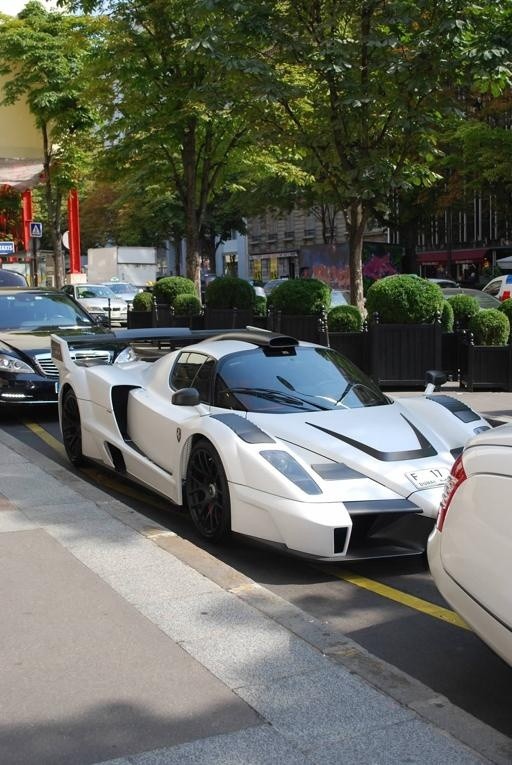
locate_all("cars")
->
[427,420,512,667]
[200,273,369,331]
[428,275,512,314]
[0,270,144,424]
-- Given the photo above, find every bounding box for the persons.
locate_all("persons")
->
[201,255,210,285]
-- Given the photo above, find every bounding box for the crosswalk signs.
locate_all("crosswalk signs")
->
[29,222,43,238]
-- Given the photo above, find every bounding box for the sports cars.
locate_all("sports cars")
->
[50,326,493,564]
